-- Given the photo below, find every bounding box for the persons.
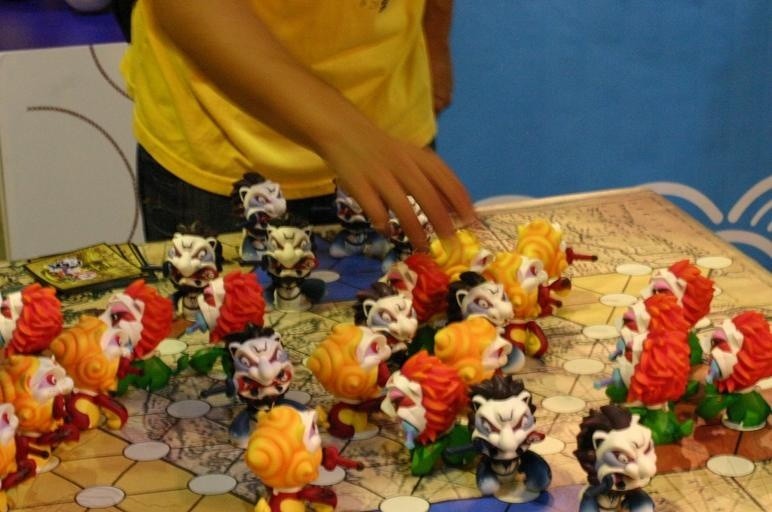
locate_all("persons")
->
[117,1,474,252]
